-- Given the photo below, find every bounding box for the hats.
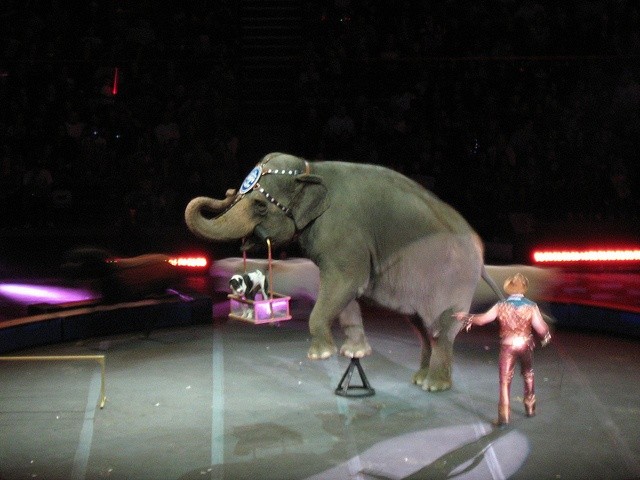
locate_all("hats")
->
[503,271,530,295]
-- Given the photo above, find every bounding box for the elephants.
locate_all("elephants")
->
[183,150,556,392]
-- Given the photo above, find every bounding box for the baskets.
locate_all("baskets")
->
[228,291,292,325]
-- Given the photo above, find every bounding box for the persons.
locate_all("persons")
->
[451,271,553,426]
